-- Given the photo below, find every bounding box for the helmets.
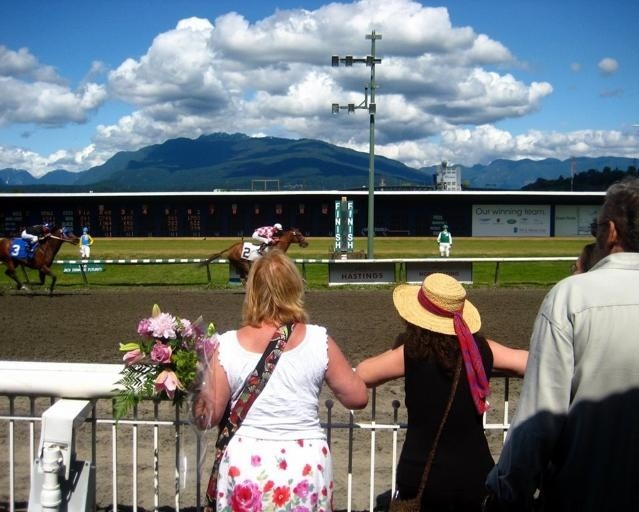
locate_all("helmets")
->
[274,223,283,231]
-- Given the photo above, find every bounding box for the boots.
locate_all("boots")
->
[258,243,266,255]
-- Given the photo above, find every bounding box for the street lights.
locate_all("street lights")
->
[328,27,384,259]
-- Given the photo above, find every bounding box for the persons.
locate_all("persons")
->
[190,250,372,510]
[478,382,638,508]
[78,227,94,259]
[434,224,454,256]
[505,179,638,449]
[353,272,531,511]
[252,223,283,254]
[21,221,53,253]
[568,212,610,276]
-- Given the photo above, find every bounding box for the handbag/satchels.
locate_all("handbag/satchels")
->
[390,496,421,510]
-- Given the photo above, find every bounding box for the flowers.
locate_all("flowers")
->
[104,298,222,428]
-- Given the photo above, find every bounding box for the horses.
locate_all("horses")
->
[195,226,309,289]
[0,225,80,296]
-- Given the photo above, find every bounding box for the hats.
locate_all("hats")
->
[393,273,481,336]
